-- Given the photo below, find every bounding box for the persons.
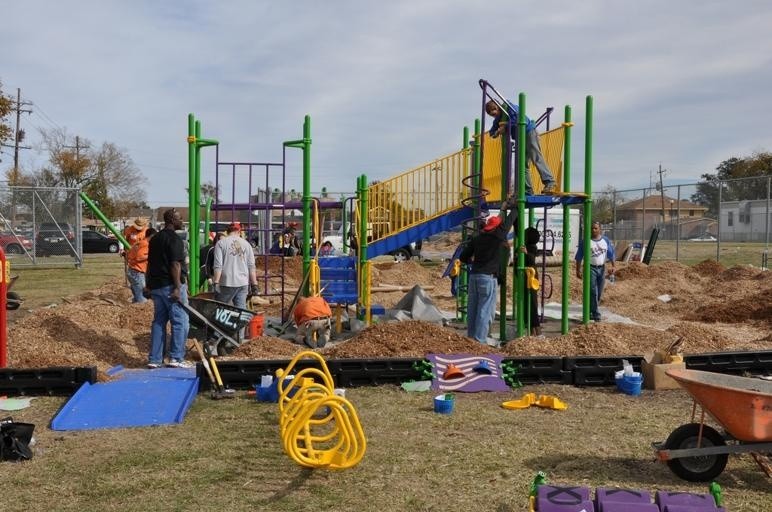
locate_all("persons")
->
[459,198,518,347]
[143,208,195,369]
[480,201,513,287]
[575,222,616,321]
[122,216,334,303]
[515,227,540,335]
[205,222,258,356]
[486,99,556,196]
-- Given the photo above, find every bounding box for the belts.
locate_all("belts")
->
[312,317,327,320]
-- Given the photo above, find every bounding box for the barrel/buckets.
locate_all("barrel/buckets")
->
[244,315,265,338]
[433,395,455,414]
[281,375,300,402]
[613,371,646,396]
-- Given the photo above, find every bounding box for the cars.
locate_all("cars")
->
[690,234,716,243]
[0,215,424,265]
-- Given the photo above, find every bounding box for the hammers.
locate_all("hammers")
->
[193,337,235,399]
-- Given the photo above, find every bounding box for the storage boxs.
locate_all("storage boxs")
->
[639,359,687,392]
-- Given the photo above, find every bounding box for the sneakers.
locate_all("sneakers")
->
[149,363,162,368]
[167,358,192,368]
[304,336,315,348]
[542,185,556,194]
[317,328,326,347]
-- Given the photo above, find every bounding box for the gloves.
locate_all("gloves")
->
[252,285,258,295]
[214,282,221,293]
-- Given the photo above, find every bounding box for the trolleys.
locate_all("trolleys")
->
[652,362,772,488]
[137,281,259,363]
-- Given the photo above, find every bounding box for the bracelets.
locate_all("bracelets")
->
[499,209,506,212]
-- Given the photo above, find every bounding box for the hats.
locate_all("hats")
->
[229,223,241,229]
[483,217,503,231]
[132,217,147,231]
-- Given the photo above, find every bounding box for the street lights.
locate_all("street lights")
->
[668,199,676,241]
